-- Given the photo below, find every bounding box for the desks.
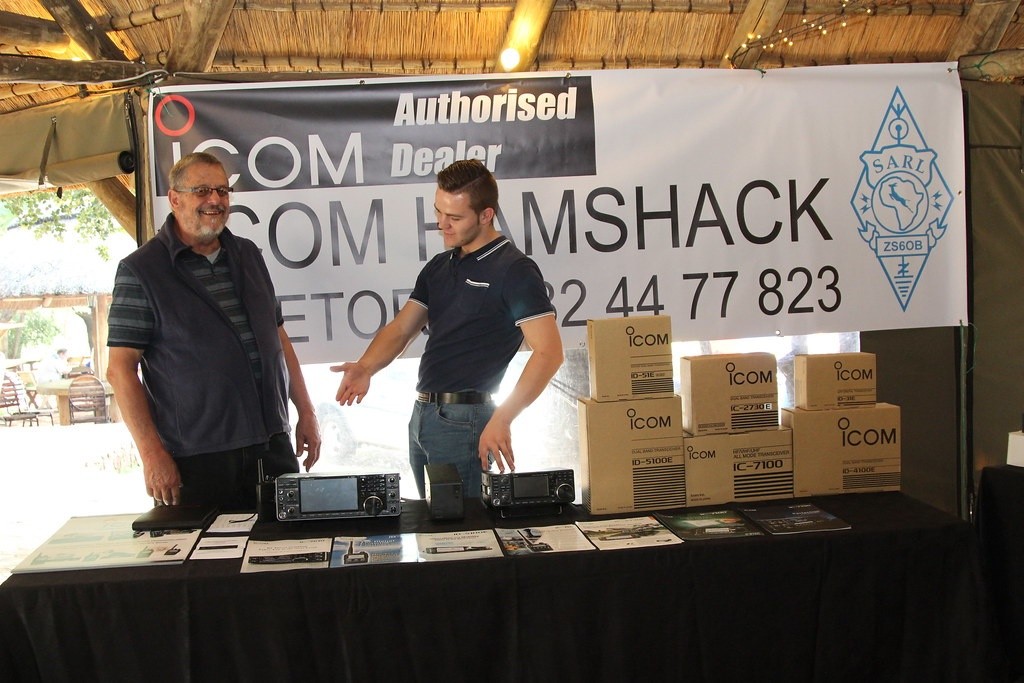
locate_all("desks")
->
[0,492,982,683]
[36,380,115,426]
[25,358,41,371]
[984,465,1024,683]
[0,359,22,373]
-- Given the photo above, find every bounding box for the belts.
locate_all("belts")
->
[418,391,491,405]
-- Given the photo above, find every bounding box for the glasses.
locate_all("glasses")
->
[175,185,234,198]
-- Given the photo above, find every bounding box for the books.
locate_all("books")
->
[737,502,852,535]
[653,509,763,540]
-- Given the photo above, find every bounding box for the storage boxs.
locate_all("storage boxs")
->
[794,352,876,410]
[781,402,901,497]
[682,425,793,507]
[578,393,686,515]
[587,315,674,403]
[680,352,778,437]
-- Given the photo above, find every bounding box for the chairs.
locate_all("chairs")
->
[0,352,5,359]
[0,372,54,428]
[68,375,107,425]
[68,366,95,379]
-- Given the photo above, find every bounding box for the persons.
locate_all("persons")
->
[105,152,321,507]
[40,348,73,381]
[331,160,564,514]
